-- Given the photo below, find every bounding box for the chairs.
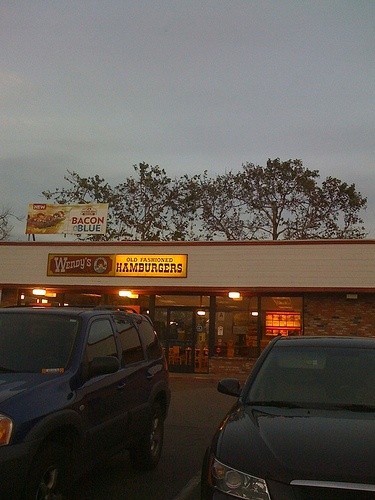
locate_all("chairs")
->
[162,345,208,368]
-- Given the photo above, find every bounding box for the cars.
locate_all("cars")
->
[201,335,375,500]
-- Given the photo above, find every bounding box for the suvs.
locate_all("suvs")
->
[0,303,172,500]
